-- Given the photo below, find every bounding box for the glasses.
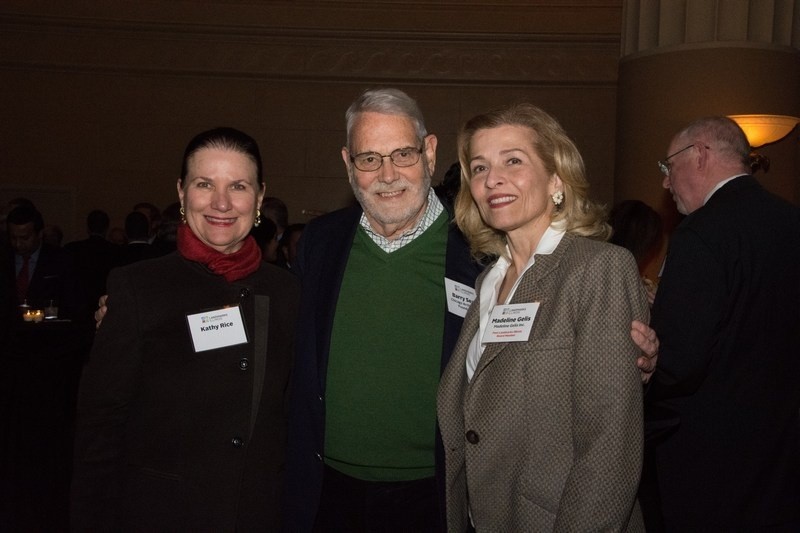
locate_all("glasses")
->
[349,141,425,172]
[654,144,710,176]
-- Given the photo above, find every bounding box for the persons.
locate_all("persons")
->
[593,198,665,326]
[0,157,497,363]
[65,125,320,533]
[638,114,800,533]
[436,100,654,533]
[91,86,660,533]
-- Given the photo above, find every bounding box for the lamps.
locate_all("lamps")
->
[729,111,799,160]
